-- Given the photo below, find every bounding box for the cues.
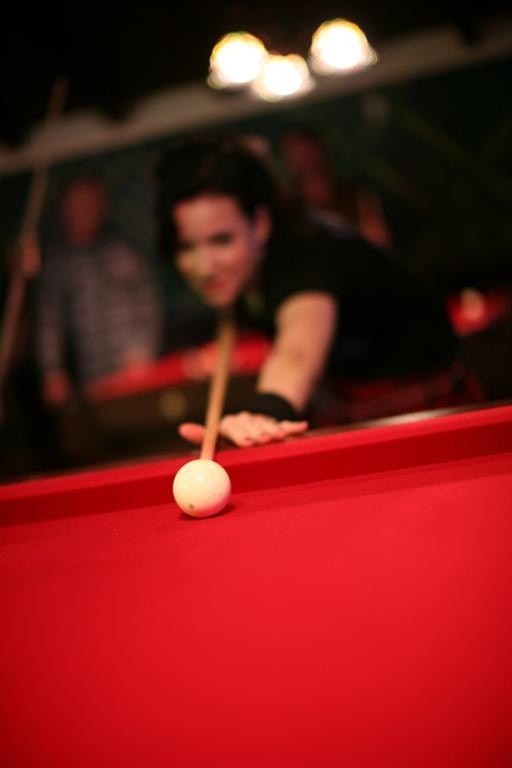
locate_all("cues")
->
[198,314,236,461]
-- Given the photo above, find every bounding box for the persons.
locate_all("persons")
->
[153,141,470,449]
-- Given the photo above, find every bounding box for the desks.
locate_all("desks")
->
[0,406,512,768]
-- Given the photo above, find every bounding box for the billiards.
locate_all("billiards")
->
[172,459,232,517]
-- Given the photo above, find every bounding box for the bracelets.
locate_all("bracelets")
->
[219,393,317,430]
